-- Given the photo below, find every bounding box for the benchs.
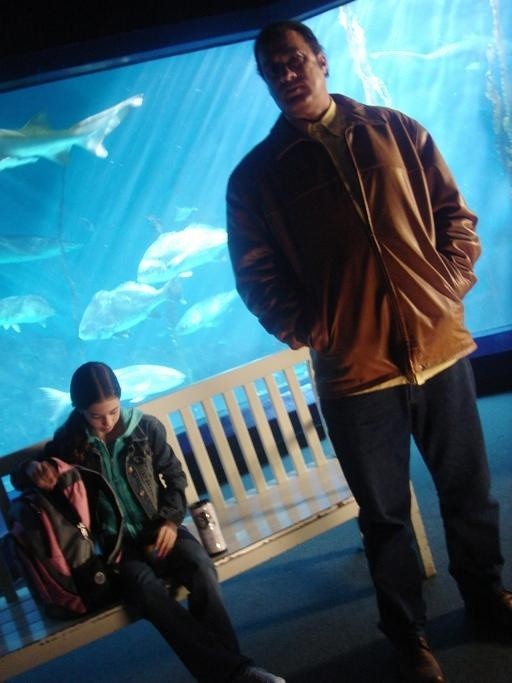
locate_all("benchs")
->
[2,342,436,677]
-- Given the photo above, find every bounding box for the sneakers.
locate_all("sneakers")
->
[463,589,512,623]
[390,631,446,683]
[244,665,286,683]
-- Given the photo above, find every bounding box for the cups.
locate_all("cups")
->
[188,498,228,557]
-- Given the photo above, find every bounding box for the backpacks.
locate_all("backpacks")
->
[10,457,125,614]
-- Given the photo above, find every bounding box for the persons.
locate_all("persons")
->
[227,20,512,683]
[10,361,285,683]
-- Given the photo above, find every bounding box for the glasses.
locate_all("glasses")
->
[264,53,320,82]
